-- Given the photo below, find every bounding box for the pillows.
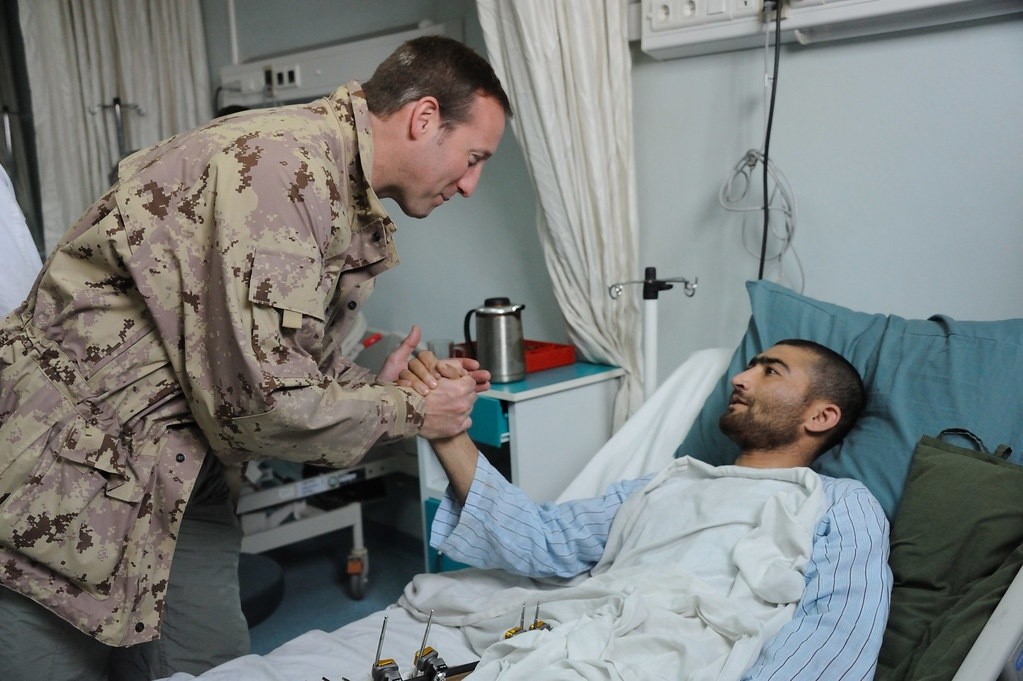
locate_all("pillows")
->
[675,280,1023,524]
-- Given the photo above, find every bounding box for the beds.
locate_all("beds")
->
[162,350,1023,680]
[236,329,425,601]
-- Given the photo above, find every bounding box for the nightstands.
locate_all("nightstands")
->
[416,362,626,576]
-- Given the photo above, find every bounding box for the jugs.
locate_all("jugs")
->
[463,297,527,383]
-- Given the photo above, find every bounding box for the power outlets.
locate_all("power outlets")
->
[220,69,268,97]
[648,0,789,31]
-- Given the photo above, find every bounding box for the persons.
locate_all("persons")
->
[393,339,893,681]
[0,34,514,681]
